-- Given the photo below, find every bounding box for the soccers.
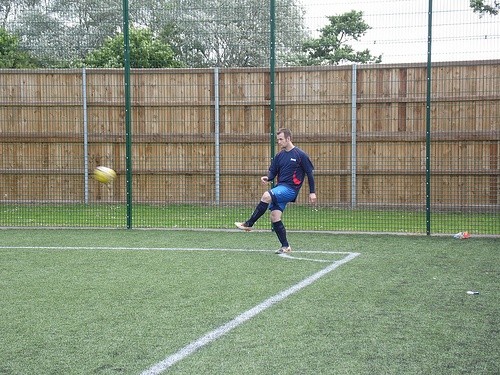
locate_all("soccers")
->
[93,166,117,184]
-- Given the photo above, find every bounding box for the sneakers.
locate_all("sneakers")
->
[235,222,252,231]
[275,246,291,253]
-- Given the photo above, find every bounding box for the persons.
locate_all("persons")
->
[234,129,317,253]
